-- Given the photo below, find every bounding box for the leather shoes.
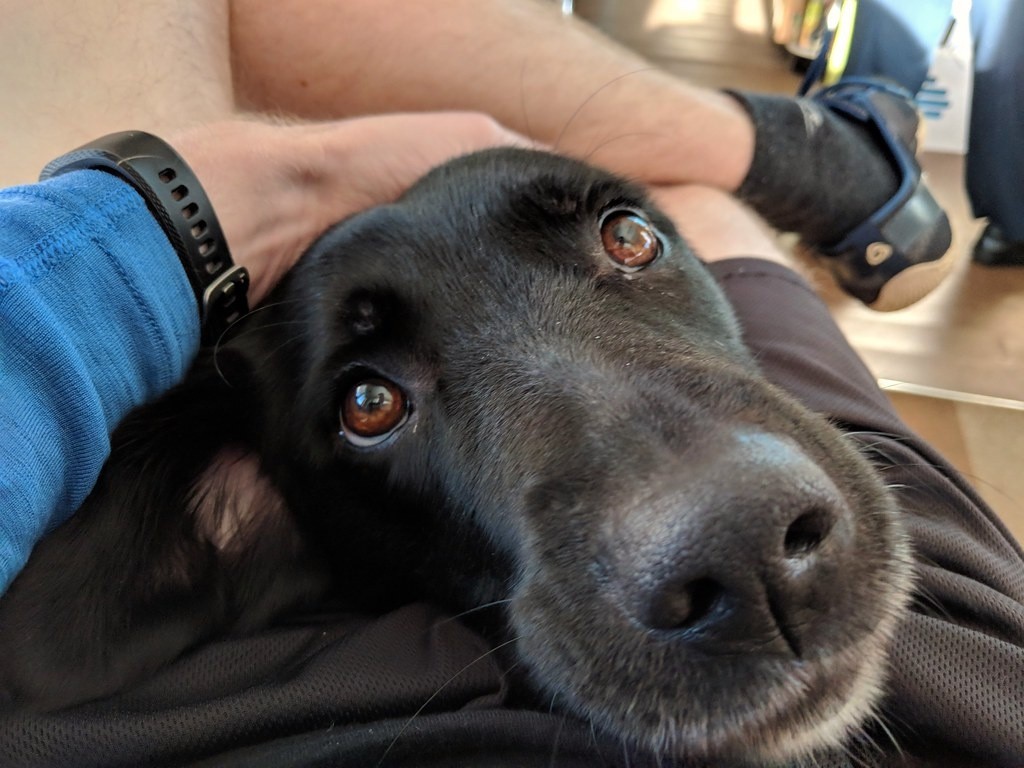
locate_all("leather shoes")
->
[973,224,1023,266]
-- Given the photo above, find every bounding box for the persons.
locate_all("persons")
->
[834,0,1024,267]
[2,1,1024,768]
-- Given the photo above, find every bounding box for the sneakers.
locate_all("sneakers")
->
[814,76,956,313]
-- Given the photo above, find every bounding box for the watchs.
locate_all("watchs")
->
[36,128,251,346]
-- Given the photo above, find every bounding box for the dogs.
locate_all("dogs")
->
[61,144,918,768]
